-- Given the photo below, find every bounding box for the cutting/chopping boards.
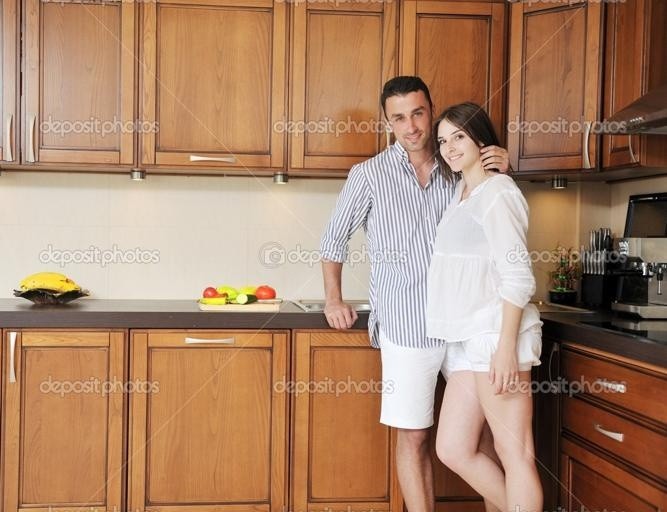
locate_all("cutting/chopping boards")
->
[199,300,281,313]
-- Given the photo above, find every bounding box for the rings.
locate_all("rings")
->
[509,381,514,385]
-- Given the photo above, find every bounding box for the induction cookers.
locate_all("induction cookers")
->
[578,313,667,346]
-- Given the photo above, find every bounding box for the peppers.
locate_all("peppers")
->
[197,297,229,305]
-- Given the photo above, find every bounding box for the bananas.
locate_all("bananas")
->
[19,272,80,293]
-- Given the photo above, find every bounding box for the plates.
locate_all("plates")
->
[13,289,90,305]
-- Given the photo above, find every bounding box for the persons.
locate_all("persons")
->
[422,102,543,512]
[322,74,517,512]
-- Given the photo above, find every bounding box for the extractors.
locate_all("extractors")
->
[590,0,667,139]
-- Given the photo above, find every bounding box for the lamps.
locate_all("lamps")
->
[131,171,145,180]
[273,174,289,184]
[551,180,567,189]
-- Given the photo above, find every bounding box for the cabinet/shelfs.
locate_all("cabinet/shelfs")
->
[506,0,666,181]
[1,327,127,512]
[560,342,667,512]
[128,329,485,511]
[1,1,506,176]
[532,332,560,512]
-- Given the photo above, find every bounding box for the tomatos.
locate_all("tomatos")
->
[203,287,217,298]
[256,285,276,300]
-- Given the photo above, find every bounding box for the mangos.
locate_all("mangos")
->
[240,287,259,295]
[216,286,238,298]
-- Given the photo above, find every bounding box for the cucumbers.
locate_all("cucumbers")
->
[236,294,257,305]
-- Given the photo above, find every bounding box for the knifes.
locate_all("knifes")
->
[582,227,617,276]
